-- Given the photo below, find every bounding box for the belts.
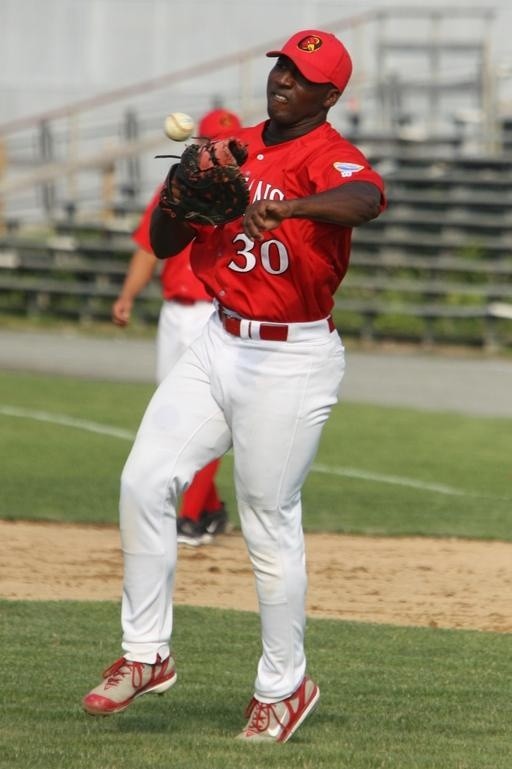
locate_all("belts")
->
[172,295,215,307]
[219,305,336,342]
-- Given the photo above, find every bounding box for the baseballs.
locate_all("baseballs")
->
[163,112,194,141]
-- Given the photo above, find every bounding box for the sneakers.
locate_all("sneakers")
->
[176,503,228,546]
[232,672,319,744]
[84,649,177,718]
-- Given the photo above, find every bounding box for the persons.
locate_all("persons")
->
[106,106,246,548]
[81,28,391,747]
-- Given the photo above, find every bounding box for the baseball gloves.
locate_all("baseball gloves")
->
[149,137,251,262]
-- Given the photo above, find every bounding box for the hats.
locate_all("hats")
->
[200,110,240,140]
[266,29,352,95]
